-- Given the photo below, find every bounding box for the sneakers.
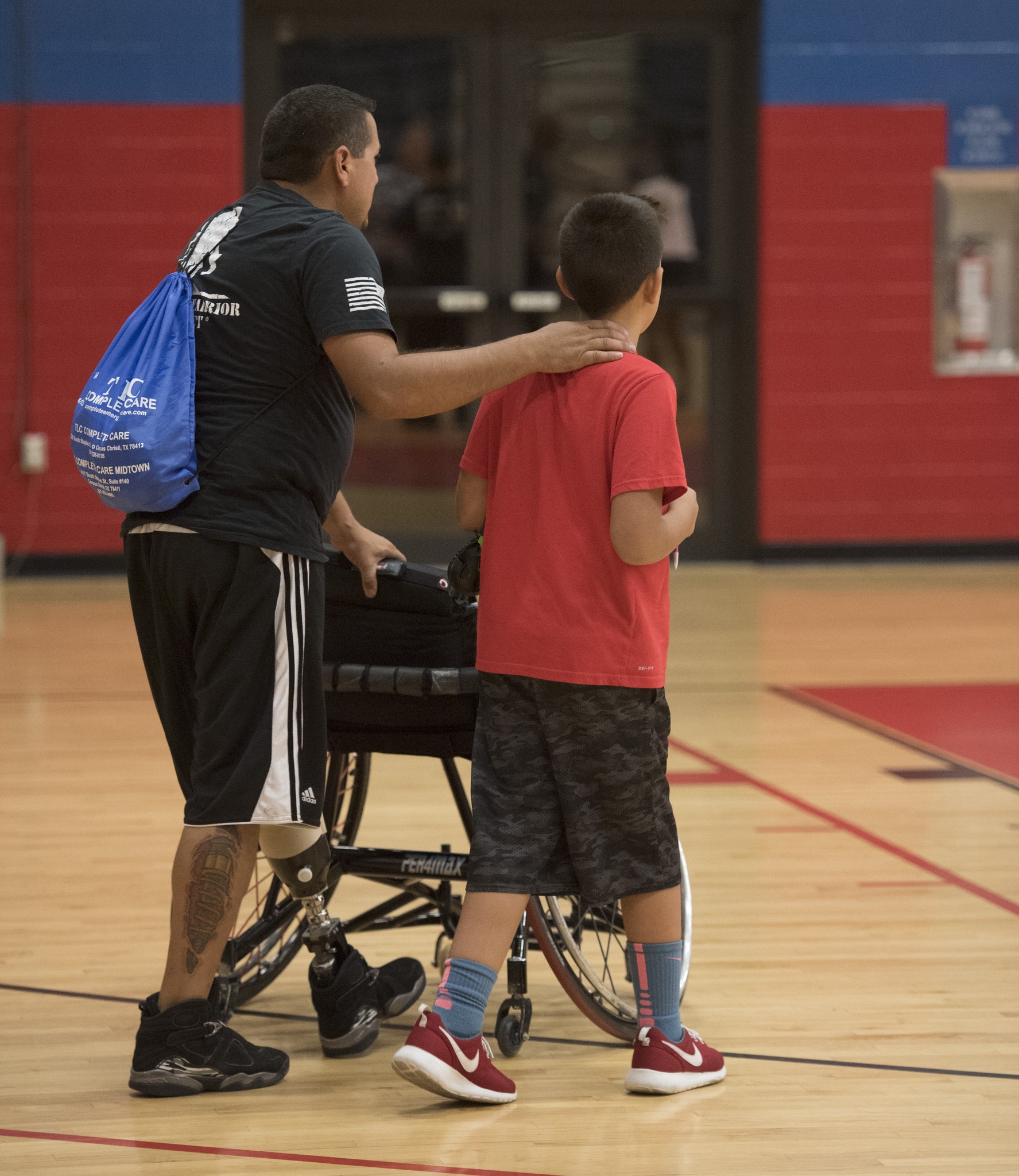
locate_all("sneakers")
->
[129,992,289,1097]
[391,1003,517,1103]
[308,944,426,1059]
[624,1024,726,1094]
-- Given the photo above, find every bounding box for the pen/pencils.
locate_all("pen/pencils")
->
[673,546,678,571]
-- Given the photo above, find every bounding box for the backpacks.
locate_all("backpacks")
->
[71,202,327,513]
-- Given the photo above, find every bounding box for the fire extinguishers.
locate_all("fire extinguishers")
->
[956,236,992,350]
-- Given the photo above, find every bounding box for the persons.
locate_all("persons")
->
[362,111,474,428]
[629,144,714,450]
[389,193,729,1104]
[120,84,637,1099]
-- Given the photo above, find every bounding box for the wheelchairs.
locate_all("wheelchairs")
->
[220,543,696,1059]
